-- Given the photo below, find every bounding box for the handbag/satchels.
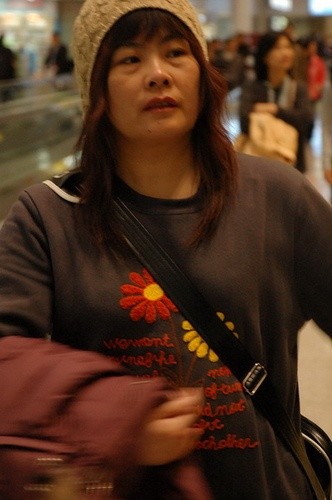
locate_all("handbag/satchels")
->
[301,413,332,500]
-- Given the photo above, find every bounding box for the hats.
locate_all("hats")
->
[71,1,209,122]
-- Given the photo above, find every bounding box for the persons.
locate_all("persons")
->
[0,1,332,500]
[204,28,332,204]
[0,28,76,108]
